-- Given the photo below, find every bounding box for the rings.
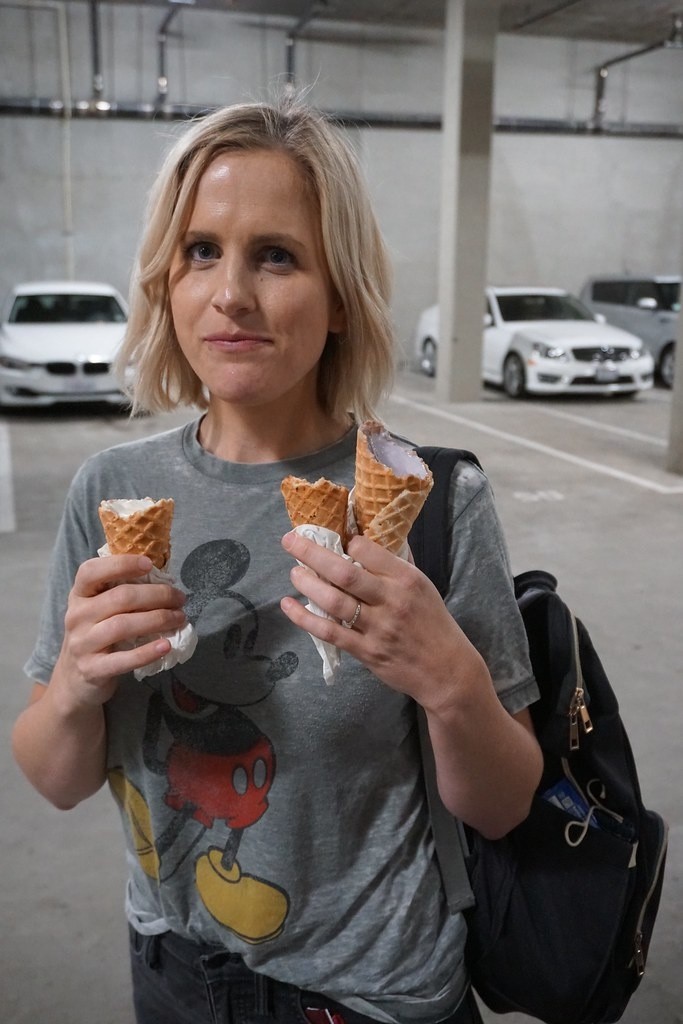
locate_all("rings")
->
[342,600,361,629]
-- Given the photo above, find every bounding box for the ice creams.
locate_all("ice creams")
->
[99,496,174,571]
[279,475,349,550]
[352,420,434,556]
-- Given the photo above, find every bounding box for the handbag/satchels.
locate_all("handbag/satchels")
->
[410,447,669,1024]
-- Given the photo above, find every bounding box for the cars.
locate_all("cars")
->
[0,279,131,412]
[582,274,682,387]
[414,286,655,401]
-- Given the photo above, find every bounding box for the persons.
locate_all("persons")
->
[10,100,544,1024]
